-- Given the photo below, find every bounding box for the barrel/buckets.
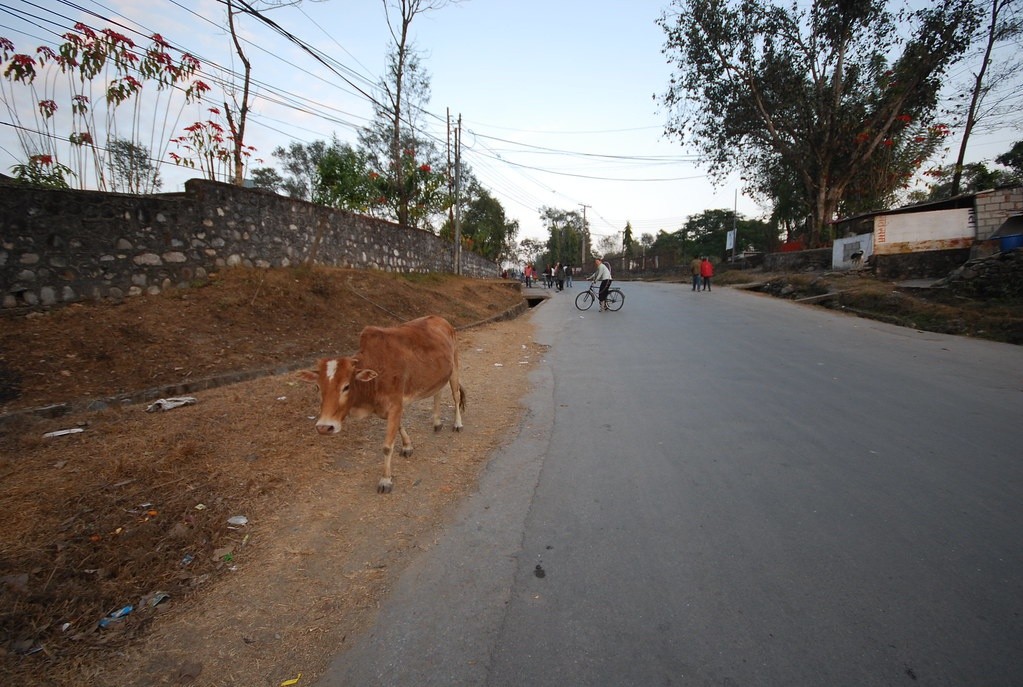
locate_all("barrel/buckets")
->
[999,233,1023,252]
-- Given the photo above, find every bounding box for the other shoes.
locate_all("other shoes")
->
[520,280,725,315]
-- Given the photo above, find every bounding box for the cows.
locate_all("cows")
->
[294,314,468,494]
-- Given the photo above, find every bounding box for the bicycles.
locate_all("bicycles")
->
[575,275,625,311]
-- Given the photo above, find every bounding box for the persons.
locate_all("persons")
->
[691,254,703,292]
[501,259,574,292]
[586,257,614,312]
[700,256,714,292]
